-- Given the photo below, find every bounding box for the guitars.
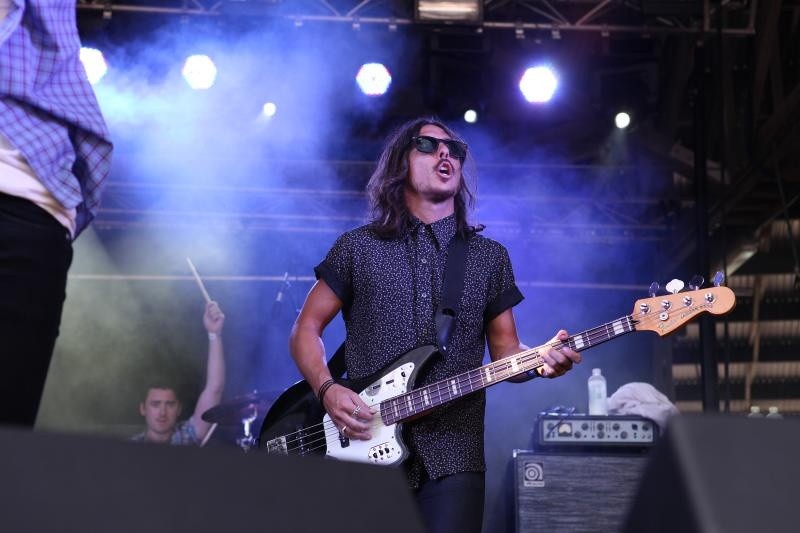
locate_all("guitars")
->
[257,268,738,469]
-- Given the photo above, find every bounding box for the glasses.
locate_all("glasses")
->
[407,136,468,165]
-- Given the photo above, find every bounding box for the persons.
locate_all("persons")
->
[126,300,226,448]
[0,0,114,432]
[289,119,582,533]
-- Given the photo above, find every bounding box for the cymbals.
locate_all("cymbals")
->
[201,386,286,428]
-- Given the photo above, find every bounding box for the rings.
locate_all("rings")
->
[353,405,361,416]
[342,425,348,434]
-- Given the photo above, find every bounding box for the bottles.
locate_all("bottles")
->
[748,407,765,418]
[767,406,785,418]
[588,368,609,416]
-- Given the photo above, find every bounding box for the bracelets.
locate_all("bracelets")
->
[208,332,222,341]
[318,379,339,411]
